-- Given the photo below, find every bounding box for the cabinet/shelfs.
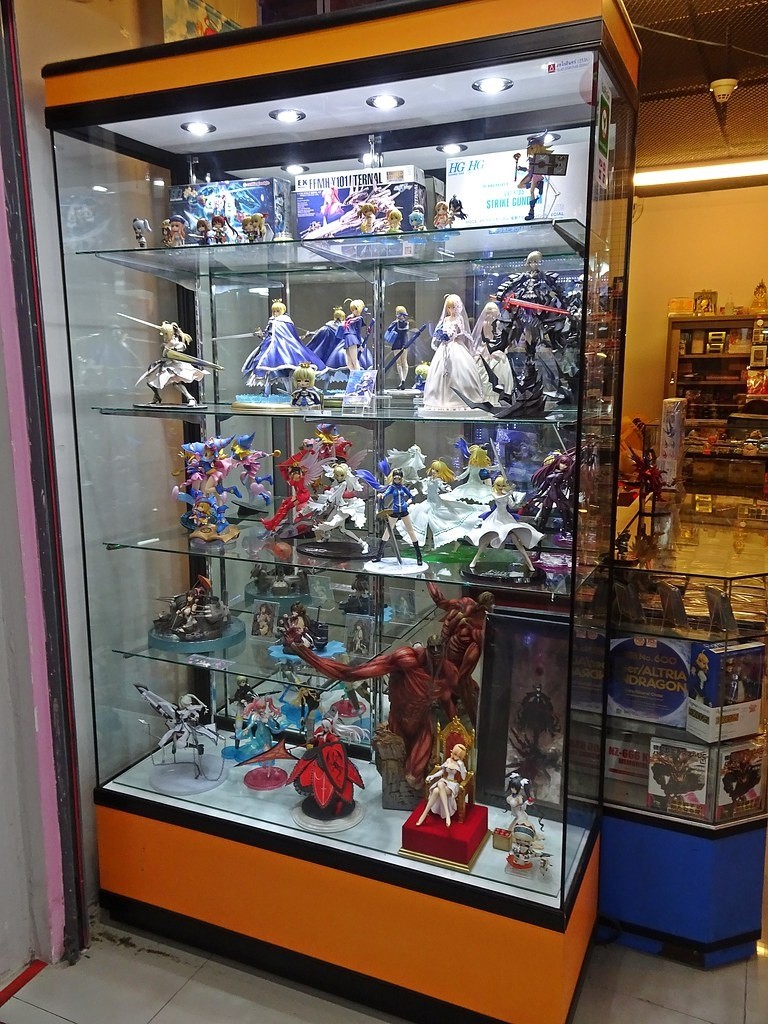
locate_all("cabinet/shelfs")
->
[662,312,768,458]
[605,491,768,971]
[41,0,645,1024]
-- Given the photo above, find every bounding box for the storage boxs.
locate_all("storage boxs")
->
[295,163,426,263]
[602,632,765,824]
[165,176,292,247]
[566,624,606,802]
[443,142,601,253]
[426,175,445,262]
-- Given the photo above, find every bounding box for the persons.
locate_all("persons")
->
[133,212,267,248]
[145,566,551,869]
[414,251,584,414]
[357,197,465,233]
[171,423,574,576]
[148,324,212,407]
[241,298,409,406]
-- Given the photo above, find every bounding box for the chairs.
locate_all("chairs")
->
[425,716,475,824]
